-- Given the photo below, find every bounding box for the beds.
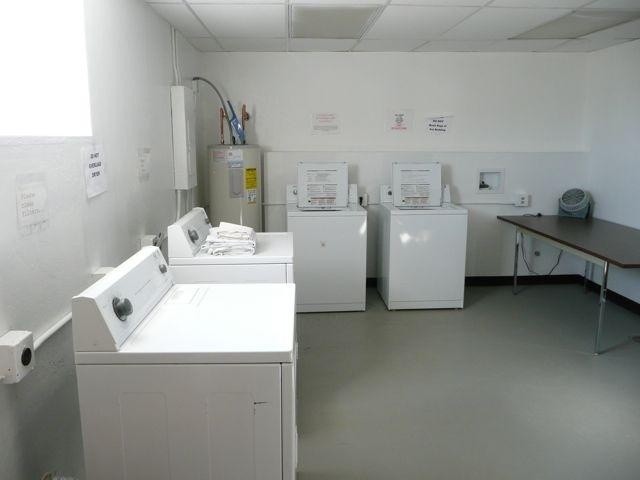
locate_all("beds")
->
[497,215,640,355]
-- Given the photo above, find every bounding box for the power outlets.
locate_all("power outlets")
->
[515,193,528,207]
